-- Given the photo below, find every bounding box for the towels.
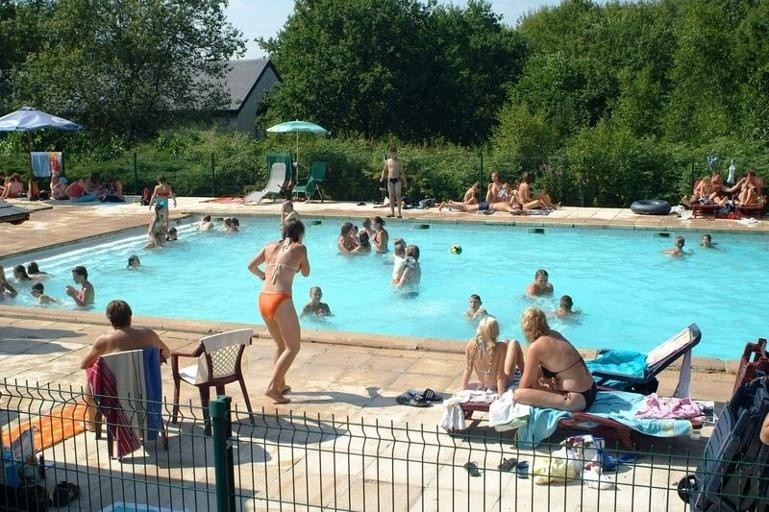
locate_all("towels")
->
[179,327,255,386]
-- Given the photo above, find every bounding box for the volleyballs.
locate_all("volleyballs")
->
[451,244,463,255]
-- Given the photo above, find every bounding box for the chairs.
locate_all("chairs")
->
[292,162,329,205]
[733,176,766,220]
[168,328,256,437]
[510,322,703,397]
[454,389,695,449]
[689,179,723,218]
[242,162,287,204]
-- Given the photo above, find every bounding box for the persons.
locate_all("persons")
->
[759,412,769,445]
[457,316,525,397]
[247,220,312,403]
[78,299,171,431]
[514,306,598,413]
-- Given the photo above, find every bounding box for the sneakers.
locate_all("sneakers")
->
[52,481,80,508]
[16,483,52,509]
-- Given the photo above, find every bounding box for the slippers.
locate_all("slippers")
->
[465,460,481,477]
[396,388,442,407]
[517,461,529,478]
[498,457,518,471]
[607,454,637,463]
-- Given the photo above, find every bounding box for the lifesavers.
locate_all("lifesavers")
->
[630,198,671,215]
[72,192,97,202]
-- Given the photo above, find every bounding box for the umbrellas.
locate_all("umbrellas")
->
[0,104,85,194]
[265,118,328,198]
